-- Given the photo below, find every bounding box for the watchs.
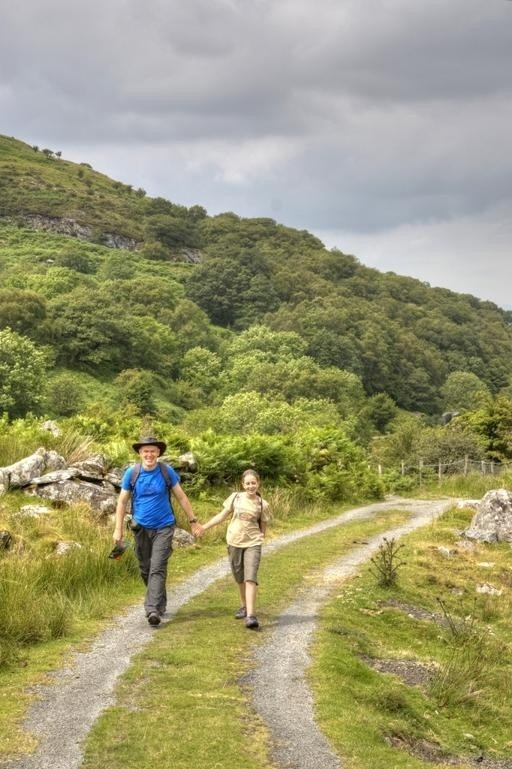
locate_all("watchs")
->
[190,518,198,524]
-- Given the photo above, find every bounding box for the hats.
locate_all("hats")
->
[132,436,166,457]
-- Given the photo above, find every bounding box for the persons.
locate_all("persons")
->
[111,438,202,625]
[195,470,271,628]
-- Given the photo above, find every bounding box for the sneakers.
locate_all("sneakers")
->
[245,615,259,628]
[147,612,161,626]
[235,602,247,618]
